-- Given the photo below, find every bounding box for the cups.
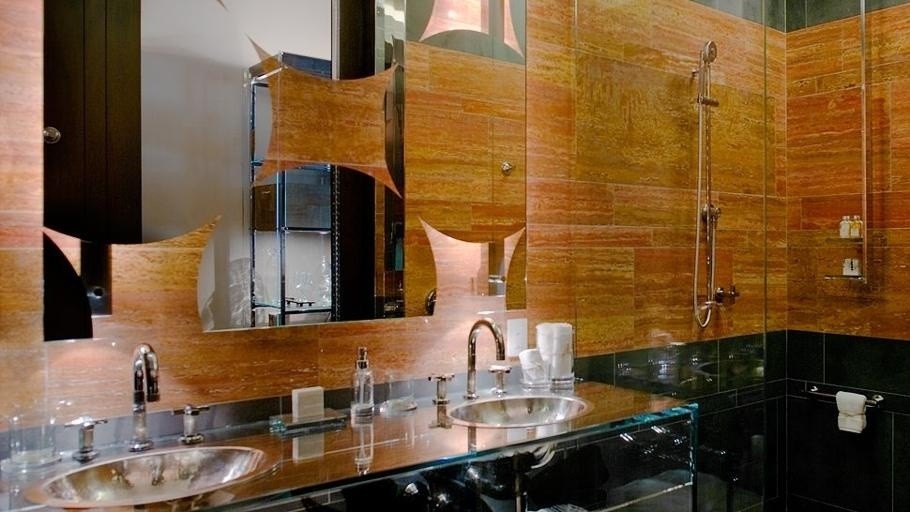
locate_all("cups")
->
[520,360,550,393]
[9,409,57,465]
[536,350,575,387]
[383,372,415,412]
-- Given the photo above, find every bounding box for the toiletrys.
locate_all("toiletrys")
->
[347,345,375,423]
[349,425,375,476]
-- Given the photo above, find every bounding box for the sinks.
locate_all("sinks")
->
[19,444,280,512]
[448,392,595,430]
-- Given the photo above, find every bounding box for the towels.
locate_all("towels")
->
[519,322,574,384]
[834,390,870,432]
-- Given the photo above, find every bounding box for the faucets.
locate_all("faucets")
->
[465,317,507,401]
[127,342,160,450]
[466,424,479,454]
[426,372,455,428]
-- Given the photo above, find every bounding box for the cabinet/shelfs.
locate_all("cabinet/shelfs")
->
[824,230,877,287]
[229,417,702,512]
[243,50,335,328]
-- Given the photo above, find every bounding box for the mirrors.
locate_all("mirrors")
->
[138,0,435,333]
